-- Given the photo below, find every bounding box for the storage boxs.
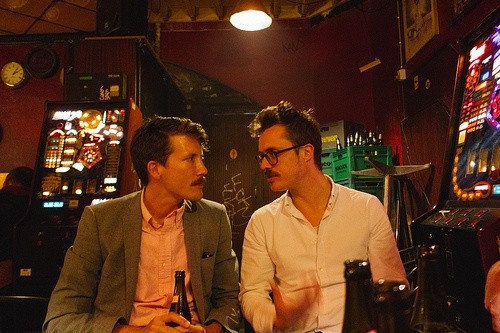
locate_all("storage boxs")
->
[318,120,395,230]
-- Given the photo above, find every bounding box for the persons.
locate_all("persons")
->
[41,116,241,333]
[238,99,412,333]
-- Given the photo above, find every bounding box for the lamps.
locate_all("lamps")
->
[229,0,272,32]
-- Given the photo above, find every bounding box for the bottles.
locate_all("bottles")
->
[342,259,414,333]
[336,137,343,158]
[347,129,384,154]
[165,270,192,327]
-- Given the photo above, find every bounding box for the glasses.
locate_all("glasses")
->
[256,145,299,166]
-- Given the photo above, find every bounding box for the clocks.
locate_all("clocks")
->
[0,60,29,88]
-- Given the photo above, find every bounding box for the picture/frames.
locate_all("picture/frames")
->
[403,0,439,64]
